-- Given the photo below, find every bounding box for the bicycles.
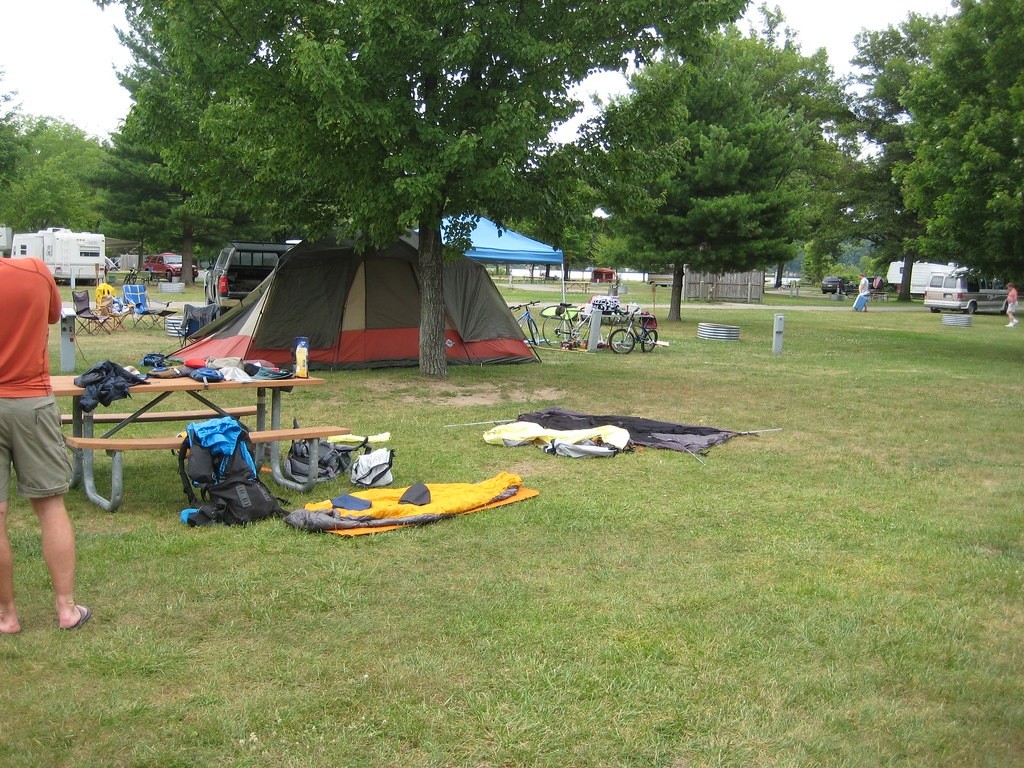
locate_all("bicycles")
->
[608,310,659,354]
[542,303,604,350]
[124,266,140,284]
[509,300,541,345]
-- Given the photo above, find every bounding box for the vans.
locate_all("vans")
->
[922,267,1009,315]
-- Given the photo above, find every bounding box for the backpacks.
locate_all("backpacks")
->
[283,438,352,483]
[176,416,292,527]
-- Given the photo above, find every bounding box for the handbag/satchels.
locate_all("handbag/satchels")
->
[350,447,397,487]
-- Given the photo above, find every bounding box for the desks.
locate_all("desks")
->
[50,374,327,502]
[853,289,889,303]
[565,281,589,294]
[512,276,555,284]
[580,313,655,345]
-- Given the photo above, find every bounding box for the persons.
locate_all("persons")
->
[1004,283,1019,327]
[0,256,91,634]
[859,274,869,312]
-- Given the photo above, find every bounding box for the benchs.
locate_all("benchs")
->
[60,406,269,490]
[66,427,351,512]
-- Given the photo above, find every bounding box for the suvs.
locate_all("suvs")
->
[205,246,279,319]
[144,253,200,283]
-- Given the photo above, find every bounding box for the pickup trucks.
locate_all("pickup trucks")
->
[852,278,884,296]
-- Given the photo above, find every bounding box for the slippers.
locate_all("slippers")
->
[60,604,92,631]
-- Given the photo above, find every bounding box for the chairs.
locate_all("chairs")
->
[71,283,220,347]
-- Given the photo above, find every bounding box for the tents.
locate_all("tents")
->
[413,212,568,340]
[165,225,542,371]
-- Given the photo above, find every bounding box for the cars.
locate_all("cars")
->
[821,276,848,295]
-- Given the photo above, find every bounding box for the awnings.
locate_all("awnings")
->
[105,237,141,264]
